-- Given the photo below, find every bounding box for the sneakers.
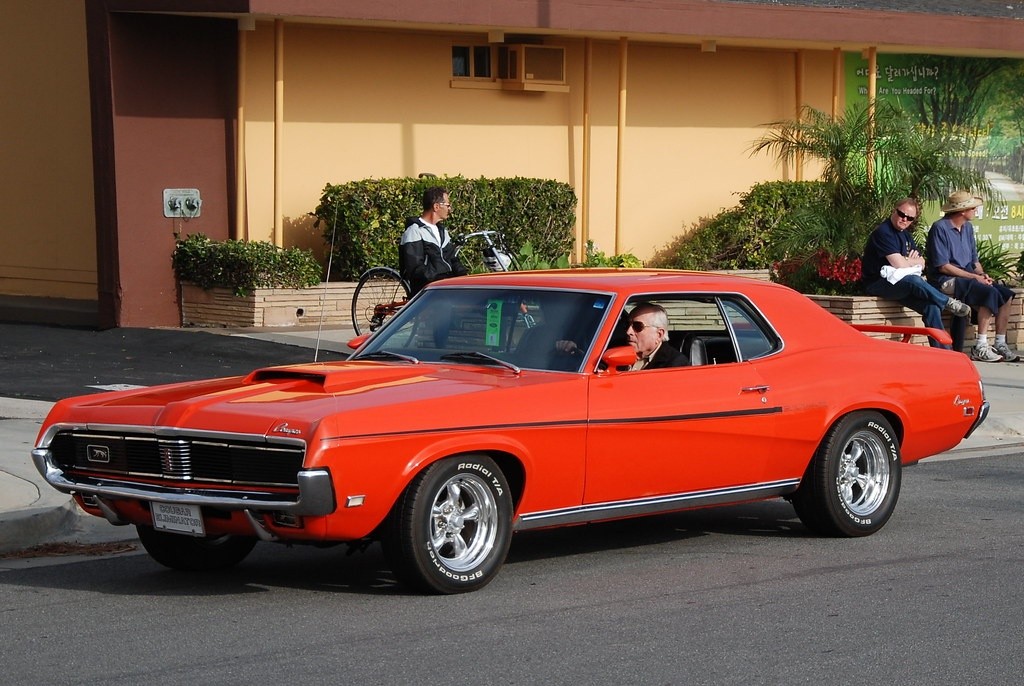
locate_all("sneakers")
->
[970,345,1004,362]
[992,344,1020,362]
[944,296,970,316]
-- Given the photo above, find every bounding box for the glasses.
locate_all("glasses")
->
[970,207,976,210]
[436,202,451,208]
[895,208,915,221]
[627,321,660,332]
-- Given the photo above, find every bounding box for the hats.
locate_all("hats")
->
[941,191,982,211]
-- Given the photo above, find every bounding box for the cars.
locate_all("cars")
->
[30,268,989,593]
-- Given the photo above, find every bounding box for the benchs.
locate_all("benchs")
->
[694,335,770,362]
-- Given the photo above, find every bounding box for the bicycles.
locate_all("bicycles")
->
[352,229,535,354]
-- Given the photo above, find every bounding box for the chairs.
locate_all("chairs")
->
[669,333,708,367]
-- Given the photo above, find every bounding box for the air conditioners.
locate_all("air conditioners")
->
[499,44,567,86]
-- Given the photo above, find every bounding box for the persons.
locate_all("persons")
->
[862,191,1020,362]
[398,186,470,349]
[556,303,691,371]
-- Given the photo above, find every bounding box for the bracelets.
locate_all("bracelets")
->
[979,273,985,276]
